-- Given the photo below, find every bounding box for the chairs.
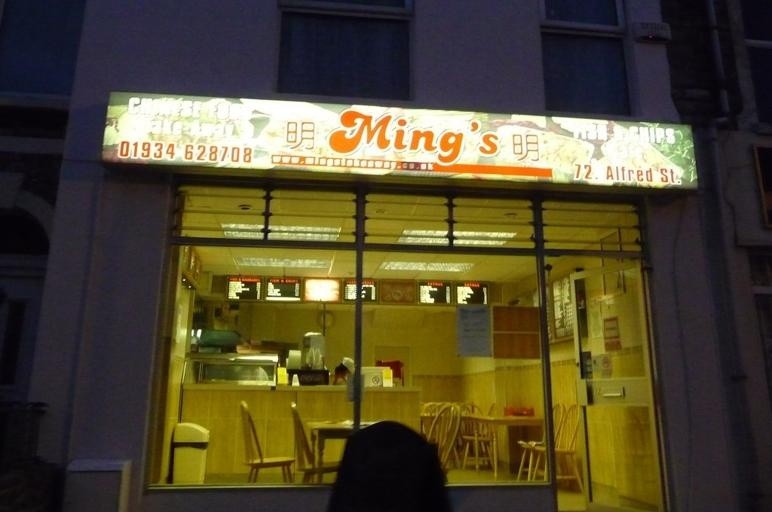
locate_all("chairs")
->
[239,399,296,485]
[516,402,584,493]
[289,402,343,486]
[418,402,498,481]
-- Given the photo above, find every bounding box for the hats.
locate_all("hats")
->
[341,357,356,373]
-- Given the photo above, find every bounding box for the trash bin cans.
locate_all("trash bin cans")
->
[171,423,210,486]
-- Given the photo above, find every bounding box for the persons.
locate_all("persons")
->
[333,357,354,385]
[324,419,457,512]
[248,364,270,381]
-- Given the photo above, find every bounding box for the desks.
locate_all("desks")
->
[305,420,376,484]
[469,414,542,482]
